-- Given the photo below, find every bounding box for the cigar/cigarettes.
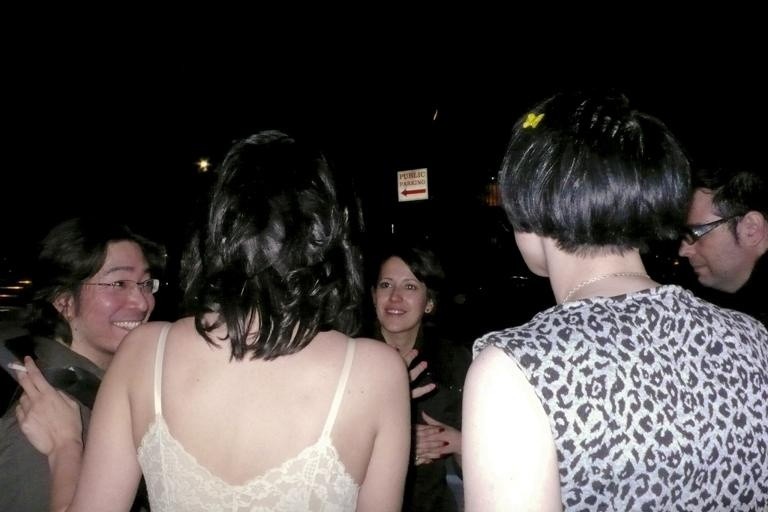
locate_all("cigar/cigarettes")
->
[6,363,27,374]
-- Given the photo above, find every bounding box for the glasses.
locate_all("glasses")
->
[78,279,160,295]
[682,213,740,245]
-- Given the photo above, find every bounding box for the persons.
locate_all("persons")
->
[0,214,157,511]
[11,125,414,512]
[460,86,768,512]
[368,233,464,512]
[674,143,768,326]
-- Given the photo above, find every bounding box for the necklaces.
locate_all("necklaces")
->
[558,270,651,306]
